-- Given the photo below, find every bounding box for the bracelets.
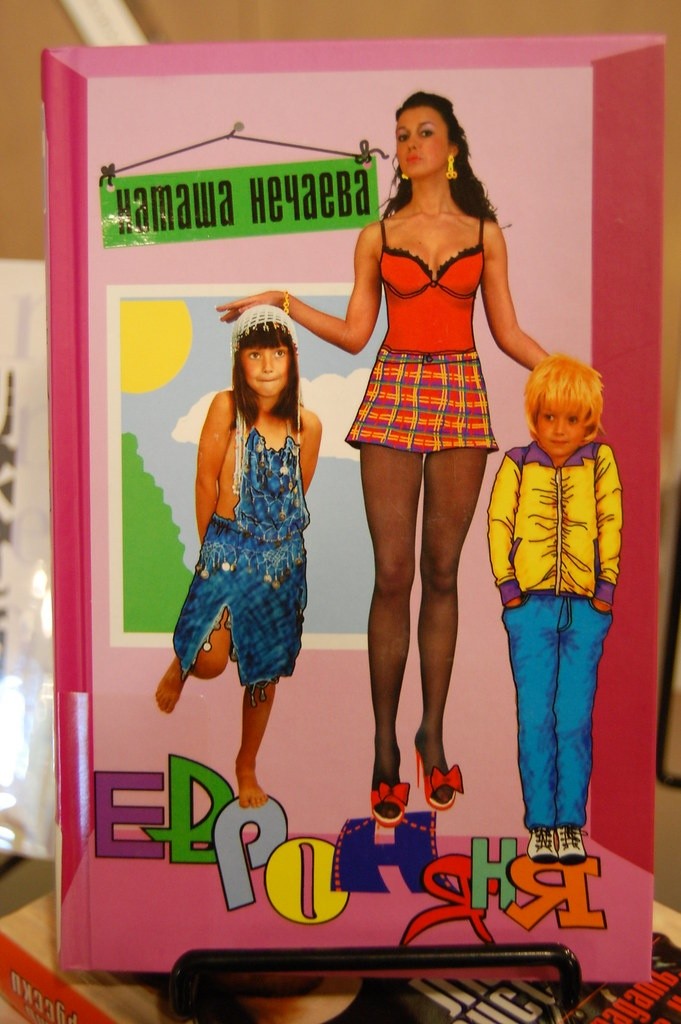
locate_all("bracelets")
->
[283,291,289,315]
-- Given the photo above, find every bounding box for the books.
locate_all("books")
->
[40,32,668,981]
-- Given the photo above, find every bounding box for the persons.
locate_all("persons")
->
[155,303,322,809]
[487,357,622,865]
[216,91,550,828]
